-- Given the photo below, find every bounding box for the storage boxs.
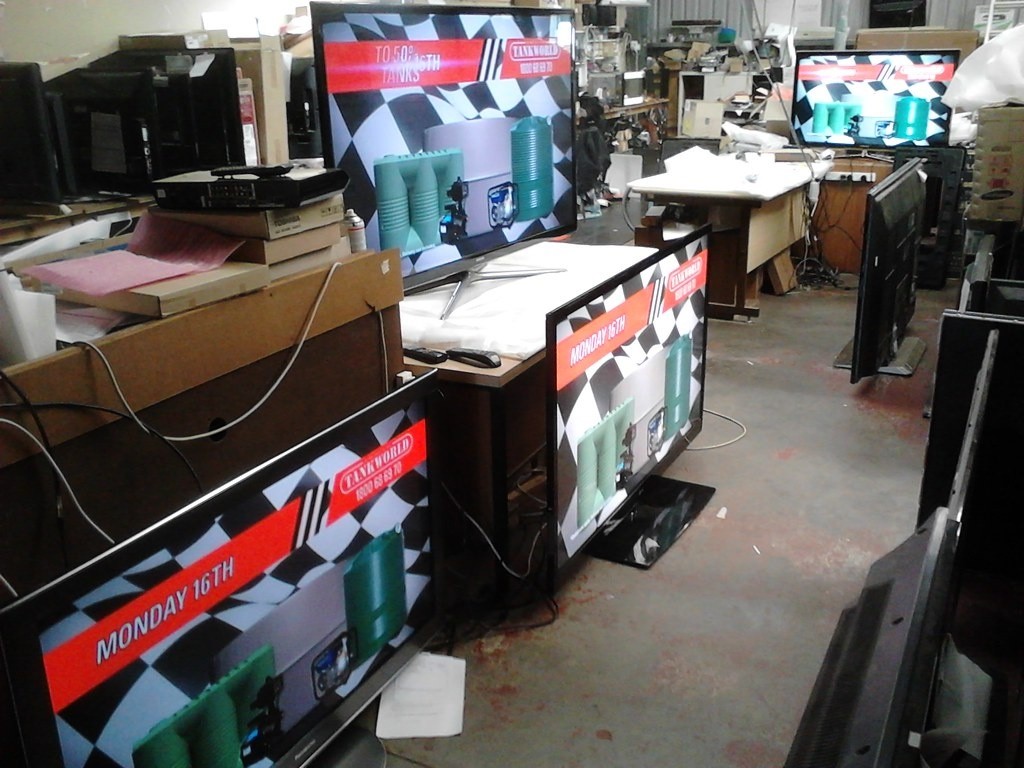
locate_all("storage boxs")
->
[31,194,351,317]
[681,99,725,139]
[855,28,980,64]
[117,25,289,166]
[969,107,1024,223]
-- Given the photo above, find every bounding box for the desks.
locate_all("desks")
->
[630,161,834,323]
[0,248,406,609]
[404,241,660,594]
[577,99,669,174]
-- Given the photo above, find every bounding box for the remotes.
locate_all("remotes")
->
[402,345,446,365]
[446,347,503,367]
[211,162,295,179]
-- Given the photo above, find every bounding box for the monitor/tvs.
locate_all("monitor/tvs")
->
[868,0,926,29]
[539,223,717,592]
[832,156,933,385]
[310,1,578,318]
[787,49,962,161]
[783,330,1003,767]
[0,47,249,220]
[0,370,443,768]
